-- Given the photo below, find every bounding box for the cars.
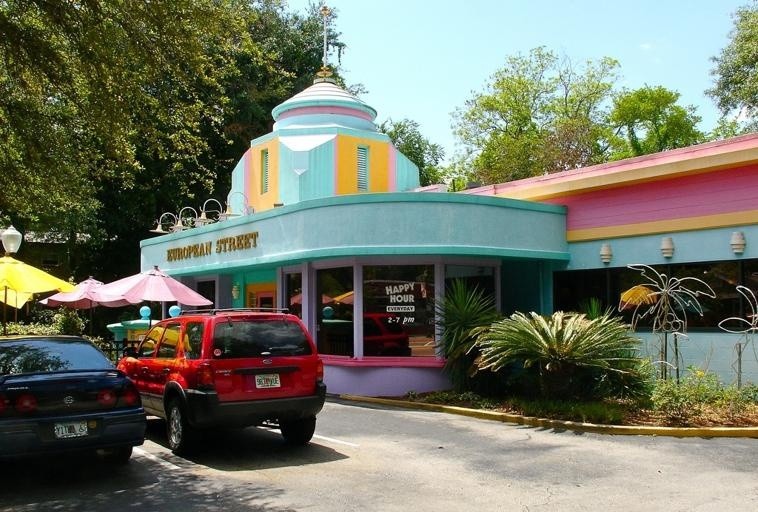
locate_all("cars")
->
[299,317,354,356]
[0,335,147,480]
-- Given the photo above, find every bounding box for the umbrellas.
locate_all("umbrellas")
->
[38,276,107,334]
[0,251,74,334]
[88,266,213,321]
[332,289,354,305]
[289,290,333,306]
[1,291,32,325]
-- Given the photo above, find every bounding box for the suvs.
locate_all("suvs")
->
[339,312,413,356]
[733,299,757,326]
[115,305,328,459]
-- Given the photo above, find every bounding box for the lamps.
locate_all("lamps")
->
[220,192,248,217]
[169,206,197,230]
[193,199,222,223]
[150,211,180,233]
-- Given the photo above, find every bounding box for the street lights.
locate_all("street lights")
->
[2,224,23,327]
